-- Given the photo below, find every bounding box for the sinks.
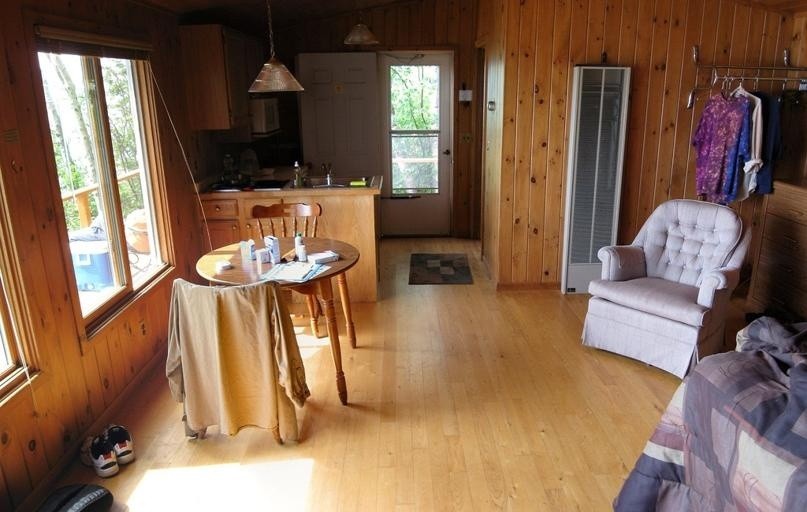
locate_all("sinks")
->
[313,183,348,188]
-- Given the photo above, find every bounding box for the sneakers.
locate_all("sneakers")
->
[81,424,135,478]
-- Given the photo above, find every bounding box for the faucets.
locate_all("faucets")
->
[320,163,333,184]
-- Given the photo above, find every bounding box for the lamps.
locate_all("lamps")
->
[344,0,382,45]
[248,0,306,93]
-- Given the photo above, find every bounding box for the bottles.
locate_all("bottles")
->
[292,159,302,188]
[293,233,308,263]
[223,154,234,178]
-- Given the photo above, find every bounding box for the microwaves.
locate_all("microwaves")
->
[248,97,281,132]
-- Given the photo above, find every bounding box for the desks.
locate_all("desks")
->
[196,236,357,406]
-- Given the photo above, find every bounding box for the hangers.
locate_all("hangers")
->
[721,75,745,95]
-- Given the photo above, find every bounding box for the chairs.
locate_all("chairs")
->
[581,199,753,380]
[251,203,323,338]
[172,278,284,447]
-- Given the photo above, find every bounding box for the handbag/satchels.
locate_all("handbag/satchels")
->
[33,484,112,512]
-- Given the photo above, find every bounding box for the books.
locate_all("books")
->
[262,247,340,284]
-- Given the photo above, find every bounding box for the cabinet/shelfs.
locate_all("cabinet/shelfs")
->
[202,198,283,251]
[181,23,244,131]
[748,183,807,320]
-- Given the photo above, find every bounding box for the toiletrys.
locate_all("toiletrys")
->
[289,161,301,188]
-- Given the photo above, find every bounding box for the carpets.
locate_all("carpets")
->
[409,253,474,285]
